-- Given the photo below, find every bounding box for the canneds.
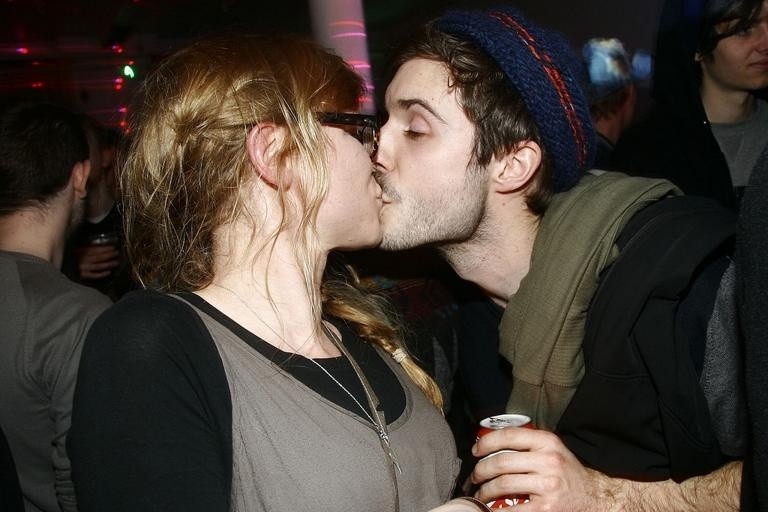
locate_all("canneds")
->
[474,412,539,512]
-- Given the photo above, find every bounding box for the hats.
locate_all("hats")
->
[428,6,598,194]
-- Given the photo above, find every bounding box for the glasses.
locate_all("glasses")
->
[312,107,389,158]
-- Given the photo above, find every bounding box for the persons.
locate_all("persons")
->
[1,1,767,511]
